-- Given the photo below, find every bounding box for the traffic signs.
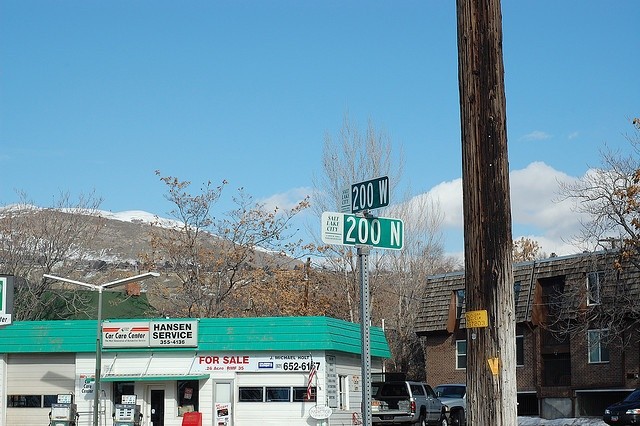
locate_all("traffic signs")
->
[320,211,404,250]
[337,176,389,213]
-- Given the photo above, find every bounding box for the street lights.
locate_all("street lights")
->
[43,272,161,426]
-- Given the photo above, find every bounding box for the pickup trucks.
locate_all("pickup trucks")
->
[360,381,450,426]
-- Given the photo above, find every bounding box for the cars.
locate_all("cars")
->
[604,388,639,426]
[432,384,467,426]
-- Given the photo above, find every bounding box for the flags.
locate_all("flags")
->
[307,356,317,400]
